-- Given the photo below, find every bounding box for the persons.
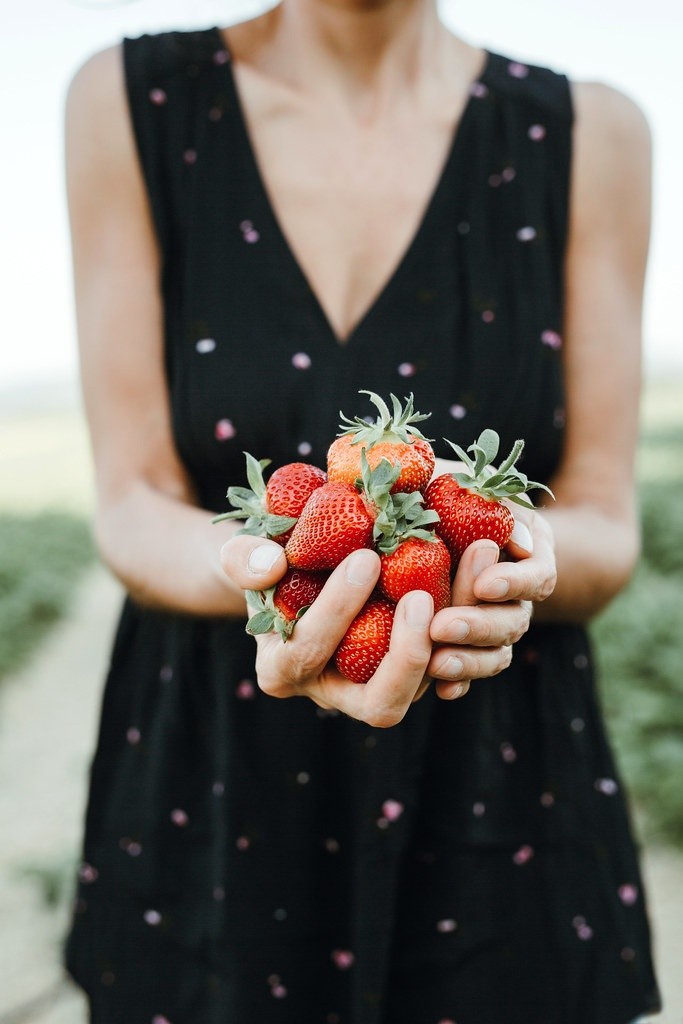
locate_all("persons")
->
[57,0,670,1023]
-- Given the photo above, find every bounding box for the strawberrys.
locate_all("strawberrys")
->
[212,391,557,688]
